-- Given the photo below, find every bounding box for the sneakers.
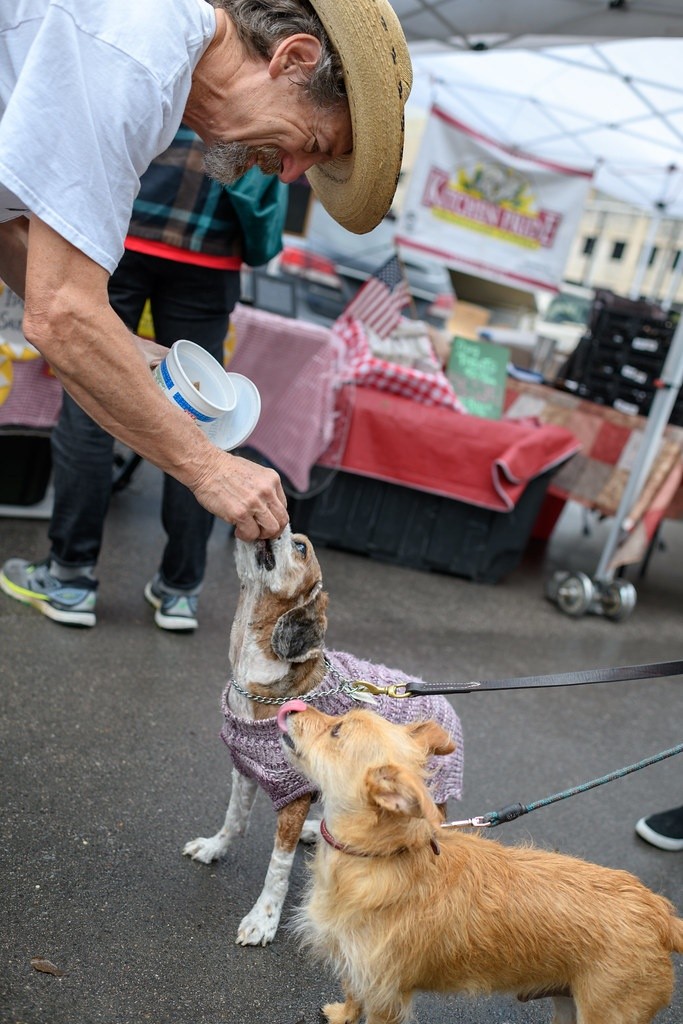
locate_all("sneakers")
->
[145,572,203,630]
[1,557,99,625]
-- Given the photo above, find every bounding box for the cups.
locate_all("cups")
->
[150,340,237,428]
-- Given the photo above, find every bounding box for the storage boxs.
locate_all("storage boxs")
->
[227,318,580,584]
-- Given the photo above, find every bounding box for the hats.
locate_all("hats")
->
[303,0,412,234]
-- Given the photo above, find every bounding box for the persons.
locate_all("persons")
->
[0,0,413,628]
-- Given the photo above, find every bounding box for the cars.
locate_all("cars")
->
[237,178,452,345]
[485,282,597,382]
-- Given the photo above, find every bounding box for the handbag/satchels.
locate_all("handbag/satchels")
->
[221,162,289,267]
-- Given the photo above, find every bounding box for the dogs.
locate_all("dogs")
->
[181,514,683,1024]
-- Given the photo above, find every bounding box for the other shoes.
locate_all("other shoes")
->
[636,804,683,852]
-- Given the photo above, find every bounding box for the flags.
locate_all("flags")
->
[344,253,414,338]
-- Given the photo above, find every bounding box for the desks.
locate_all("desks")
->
[505,373,683,589]
